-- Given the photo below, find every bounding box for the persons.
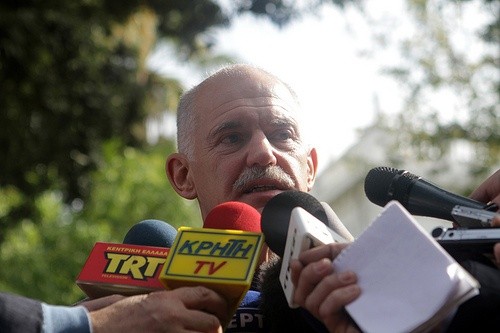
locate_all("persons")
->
[0,167,500,333]
[166,65,318,290]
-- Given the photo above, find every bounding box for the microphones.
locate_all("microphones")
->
[365,167,489,222]
[77,189,350,333]
